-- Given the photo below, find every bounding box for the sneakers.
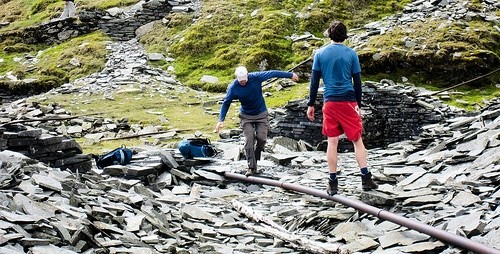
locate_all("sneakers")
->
[327,177,338,195]
[361,171,378,191]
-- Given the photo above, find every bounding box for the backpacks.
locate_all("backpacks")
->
[97,144,132,169]
[178,138,217,161]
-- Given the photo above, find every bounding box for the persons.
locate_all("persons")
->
[214,66,299,177]
[307,21,379,196]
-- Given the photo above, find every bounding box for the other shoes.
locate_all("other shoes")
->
[245,168,253,176]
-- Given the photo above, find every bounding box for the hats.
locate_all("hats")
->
[235,66,248,82]
